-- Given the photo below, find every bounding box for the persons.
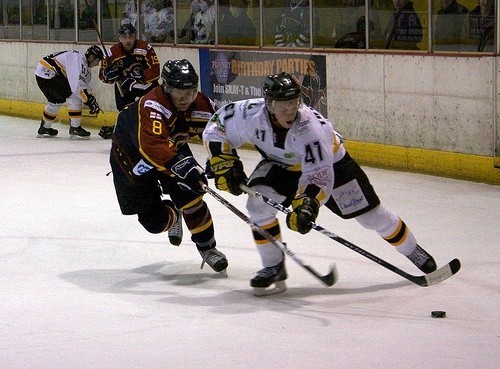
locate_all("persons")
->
[381,0,422,49]
[99,23,160,111]
[462,0,494,45]
[0,0,74,29]
[109,60,228,278]
[275,0,320,47]
[435,0,470,45]
[331,0,387,48]
[79,0,114,41]
[34,45,104,140]
[172,0,256,46]
[202,73,437,296]
[120,0,174,42]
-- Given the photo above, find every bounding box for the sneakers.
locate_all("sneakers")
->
[404,244,437,274]
[168,213,183,246]
[36,120,59,138]
[250,243,287,296]
[197,246,227,277]
[69,125,91,140]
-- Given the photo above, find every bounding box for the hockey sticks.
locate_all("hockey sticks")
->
[240,183,461,287]
[85,0,125,98]
[198,179,339,287]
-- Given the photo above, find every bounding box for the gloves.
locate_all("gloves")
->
[102,64,122,83]
[84,95,100,114]
[285,193,320,234]
[129,67,143,82]
[98,125,114,139]
[165,151,208,194]
[210,153,248,196]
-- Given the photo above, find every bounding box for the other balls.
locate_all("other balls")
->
[431,311,446,318]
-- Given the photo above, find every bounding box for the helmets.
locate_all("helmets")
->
[118,24,136,35]
[87,45,103,61]
[160,59,199,90]
[264,73,300,101]
